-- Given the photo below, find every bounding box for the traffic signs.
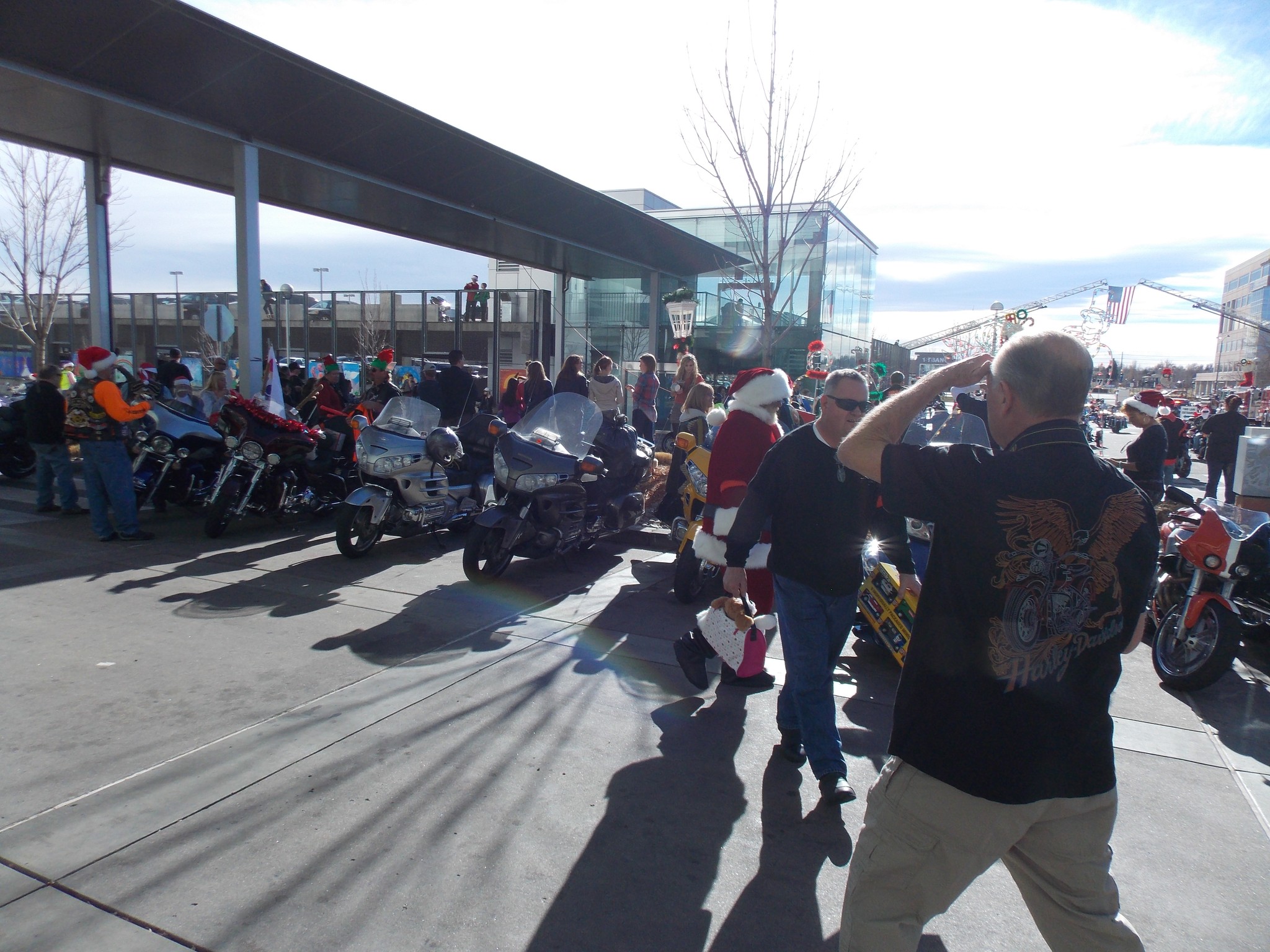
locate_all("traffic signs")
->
[1180,406,1198,421]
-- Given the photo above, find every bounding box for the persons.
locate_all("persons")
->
[474,283,490,323]
[464,275,479,322]
[24,328,1270,951]
[260,279,275,320]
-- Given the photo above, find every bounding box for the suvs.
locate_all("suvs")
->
[1163,390,1186,397]
[351,355,378,370]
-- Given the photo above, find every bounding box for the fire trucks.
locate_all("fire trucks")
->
[1215,386,1270,426]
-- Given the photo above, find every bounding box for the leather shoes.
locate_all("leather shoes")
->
[818,772,856,805]
[780,731,807,762]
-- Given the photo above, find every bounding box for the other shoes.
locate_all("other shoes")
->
[101,532,117,542]
[62,506,89,515]
[724,659,774,688]
[673,634,710,689]
[119,530,155,541]
[38,504,62,512]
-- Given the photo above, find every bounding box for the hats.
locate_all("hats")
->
[423,363,436,371]
[371,348,397,373]
[1122,391,1170,418]
[322,355,339,374]
[472,275,478,282]
[138,363,155,381]
[174,377,191,387]
[63,362,75,369]
[707,368,793,427]
[77,347,116,379]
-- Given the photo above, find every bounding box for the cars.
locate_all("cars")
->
[277,357,316,368]
[309,300,360,320]
[1173,399,1191,410]
[0,294,238,321]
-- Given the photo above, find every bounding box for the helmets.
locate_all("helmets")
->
[425,428,459,464]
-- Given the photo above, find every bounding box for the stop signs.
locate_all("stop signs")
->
[1155,384,1162,390]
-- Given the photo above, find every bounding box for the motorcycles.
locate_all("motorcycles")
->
[463,392,657,586]
[1146,483,1270,692]
[852,410,995,653]
[336,396,522,559]
[132,388,358,539]
[1087,403,1128,433]
[669,396,733,605]
[0,393,36,479]
[913,408,932,442]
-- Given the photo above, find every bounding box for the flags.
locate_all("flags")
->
[1104,285,1136,324]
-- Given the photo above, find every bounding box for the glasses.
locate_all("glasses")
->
[824,394,870,413]
[52,373,63,378]
[372,368,380,372]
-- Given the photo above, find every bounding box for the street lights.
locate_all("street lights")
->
[343,294,355,302]
[170,271,184,292]
[1214,335,1230,392]
[280,284,294,366]
[990,302,1003,356]
[313,268,329,306]
